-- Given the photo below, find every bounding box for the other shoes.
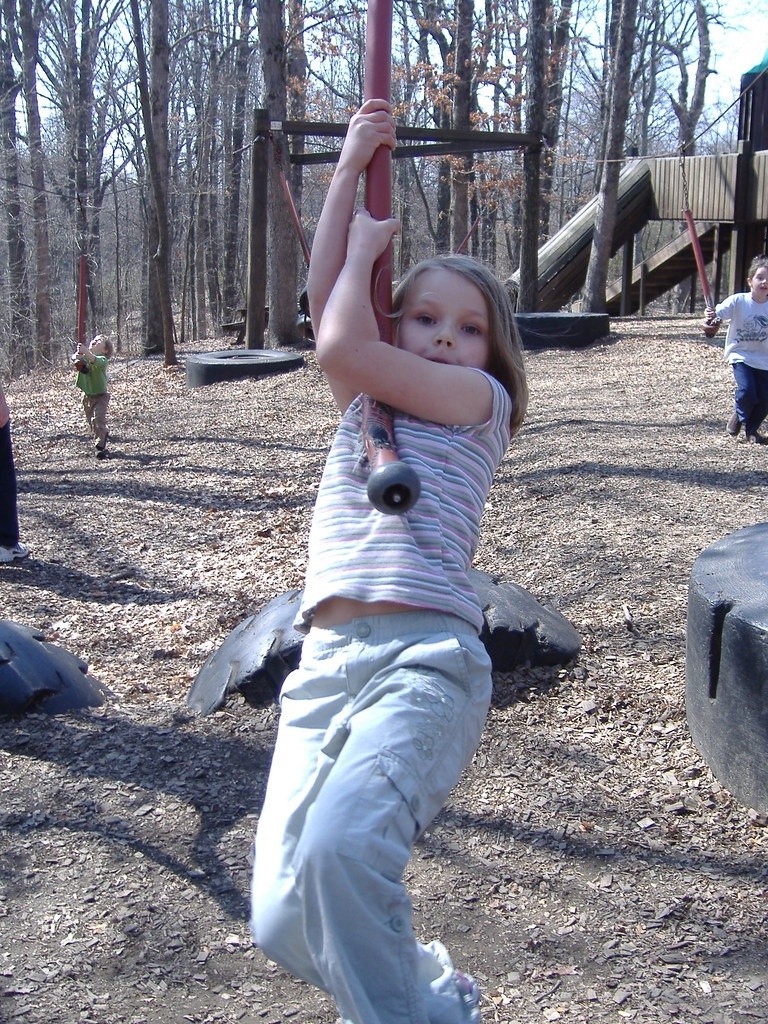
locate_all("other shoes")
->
[95,446,105,457]
[745,430,763,443]
[727,412,742,435]
[454,969,481,1024]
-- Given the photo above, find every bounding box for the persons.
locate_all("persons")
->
[0,387,30,564]
[245,96,529,1023]
[703,254,768,444]
[73,333,114,458]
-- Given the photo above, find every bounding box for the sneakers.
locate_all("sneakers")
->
[0,542,30,563]
